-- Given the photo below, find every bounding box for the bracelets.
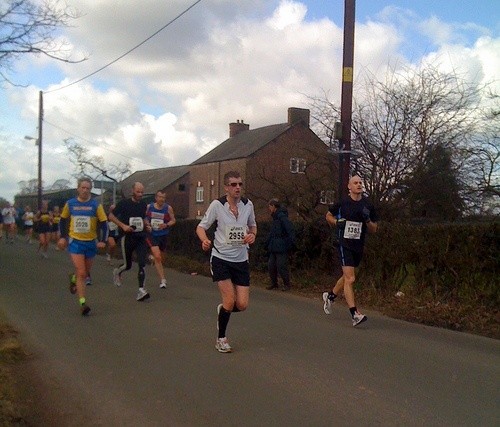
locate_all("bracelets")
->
[248,232,255,235]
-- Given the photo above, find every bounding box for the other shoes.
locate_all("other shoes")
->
[281,285,290,291]
[266,284,279,290]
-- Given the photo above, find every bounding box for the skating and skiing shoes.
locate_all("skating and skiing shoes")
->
[217,304,223,330]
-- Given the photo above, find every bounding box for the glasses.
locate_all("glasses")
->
[228,182,243,187]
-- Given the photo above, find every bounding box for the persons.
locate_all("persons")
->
[263,198,296,291]
[0,200,62,259]
[101,204,121,264]
[110,181,150,301]
[196,171,257,352]
[57,176,109,316]
[146,191,176,289]
[322,175,378,326]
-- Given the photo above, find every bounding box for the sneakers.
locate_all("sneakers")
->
[322,292,332,315]
[69,273,77,294]
[86,276,91,285]
[104,253,113,265]
[149,255,155,266]
[136,289,150,302]
[113,267,123,288]
[81,302,90,316]
[159,279,168,288]
[215,337,232,353]
[351,311,366,326]
